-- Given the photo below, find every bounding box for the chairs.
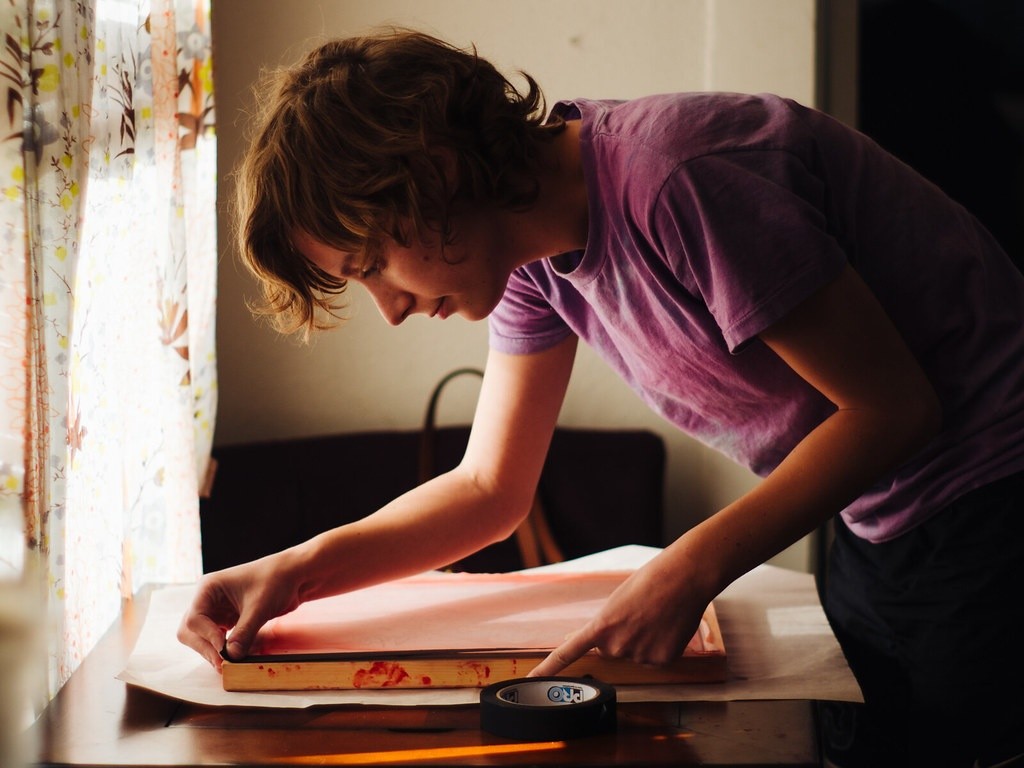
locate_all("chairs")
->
[200,426,667,574]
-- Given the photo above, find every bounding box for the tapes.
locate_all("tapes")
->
[478,676,618,741]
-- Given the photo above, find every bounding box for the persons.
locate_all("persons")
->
[178,25,1024,768]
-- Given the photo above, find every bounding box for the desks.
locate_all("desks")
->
[13,577,814,767]
